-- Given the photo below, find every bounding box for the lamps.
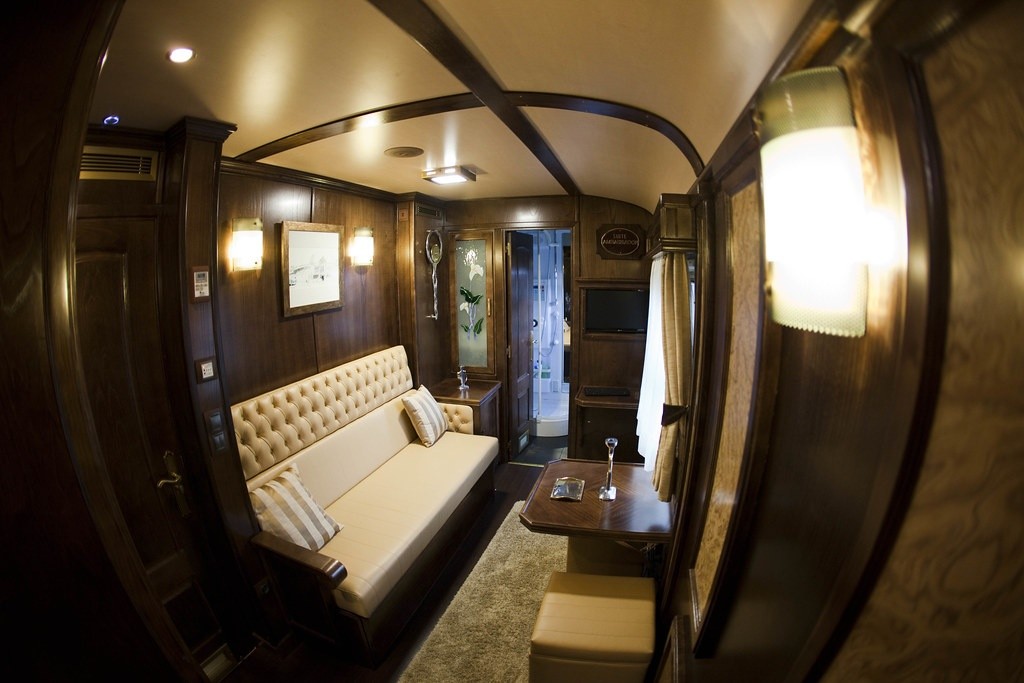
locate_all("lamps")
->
[421,166,476,185]
[354,227,373,266]
[232,218,264,271]
[753,65,867,337]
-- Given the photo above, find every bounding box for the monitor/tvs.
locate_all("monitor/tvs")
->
[585,289,648,334]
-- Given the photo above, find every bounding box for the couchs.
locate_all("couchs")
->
[230,344,499,668]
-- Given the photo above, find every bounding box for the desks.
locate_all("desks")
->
[575,385,640,409]
[519,458,676,577]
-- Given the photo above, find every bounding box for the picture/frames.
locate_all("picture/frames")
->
[281,221,345,317]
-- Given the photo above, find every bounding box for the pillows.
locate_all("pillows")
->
[248,463,344,552]
[401,384,449,447]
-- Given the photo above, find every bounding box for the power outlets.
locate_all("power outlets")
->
[201,362,213,379]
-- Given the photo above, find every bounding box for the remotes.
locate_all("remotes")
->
[584,387,630,396]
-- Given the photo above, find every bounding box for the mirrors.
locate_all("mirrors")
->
[50,0,946,683]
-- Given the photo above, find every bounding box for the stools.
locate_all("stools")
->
[529,572,655,683]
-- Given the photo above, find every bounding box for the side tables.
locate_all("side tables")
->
[430,378,502,471]
